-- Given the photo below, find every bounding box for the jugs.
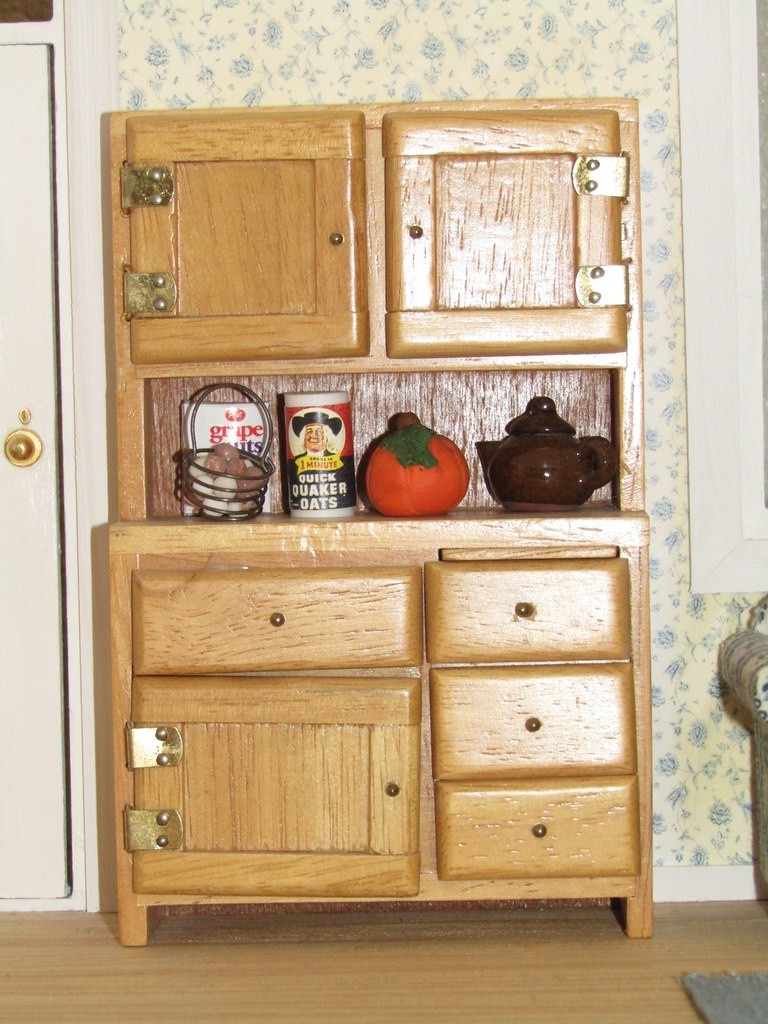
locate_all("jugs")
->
[474,396,617,512]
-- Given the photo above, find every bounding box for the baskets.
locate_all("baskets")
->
[180,382,276,520]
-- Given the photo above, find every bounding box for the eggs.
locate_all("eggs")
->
[188,443,267,519]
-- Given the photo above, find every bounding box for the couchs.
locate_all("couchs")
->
[719,594,768,882]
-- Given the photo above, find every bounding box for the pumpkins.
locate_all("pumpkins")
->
[365,422,469,517]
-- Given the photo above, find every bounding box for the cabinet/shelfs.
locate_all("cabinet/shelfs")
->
[106,98,654,948]
